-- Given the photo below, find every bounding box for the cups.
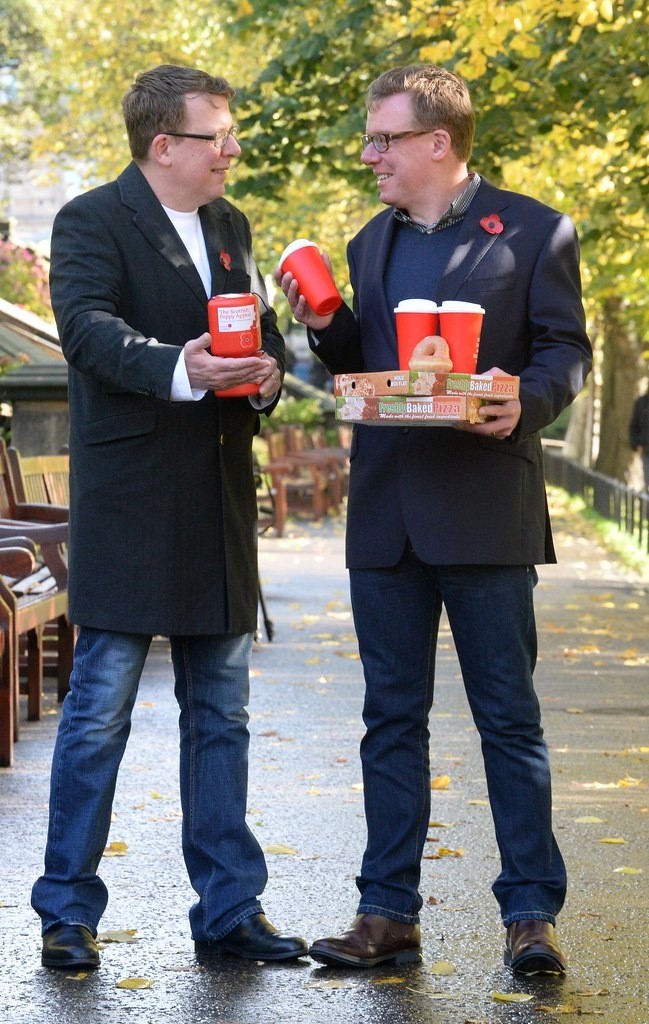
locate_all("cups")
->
[438,300,485,375]
[393,299,438,370]
[278,237,343,316]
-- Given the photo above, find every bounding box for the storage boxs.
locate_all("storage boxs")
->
[332,371,520,427]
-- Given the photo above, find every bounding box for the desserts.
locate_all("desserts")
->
[408,335,453,374]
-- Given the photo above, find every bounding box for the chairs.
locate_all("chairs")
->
[1,439,76,767]
[260,421,353,538]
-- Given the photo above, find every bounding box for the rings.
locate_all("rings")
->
[490,430,497,440]
[271,373,277,385]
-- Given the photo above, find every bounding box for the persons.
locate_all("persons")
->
[30,65,310,970]
[275,62,596,983]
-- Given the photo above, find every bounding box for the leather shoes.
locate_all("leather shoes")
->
[309,912,423,967]
[195,912,308,960]
[504,919,567,974]
[42,925,100,966]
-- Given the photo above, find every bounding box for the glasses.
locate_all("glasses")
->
[157,124,239,147]
[360,129,437,153]
